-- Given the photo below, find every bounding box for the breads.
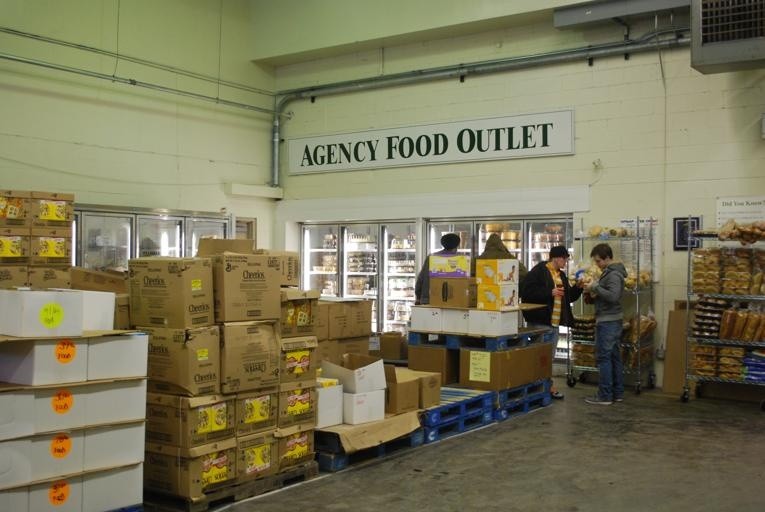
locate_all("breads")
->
[622,313,657,343]
[719,218,765,246]
[720,307,765,341]
[692,245,765,296]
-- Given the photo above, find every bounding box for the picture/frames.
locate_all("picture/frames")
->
[672,217,701,252]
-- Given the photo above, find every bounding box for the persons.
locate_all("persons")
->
[583,244,629,405]
[522,246,583,399]
[415,233,460,304]
[469,233,527,279]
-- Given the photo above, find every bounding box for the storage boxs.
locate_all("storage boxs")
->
[235,386,278,437]
[383,363,419,412]
[0,228,31,266]
[408,345,450,384]
[321,352,387,394]
[209,251,281,321]
[413,370,442,409]
[531,342,552,378]
[280,288,320,338]
[0,289,83,336]
[0,438,31,487]
[86,379,147,422]
[0,390,36,441]
[30,228,72,263]
[442,309,469,332]
[83,463,143,512]
[29,477,82,512]
[0,190,32,228]
[476,258,519,284]
[379,331,407,360]
[411,306,442,329]
[343,390,385,424]
[115,294,130,328]
[129,255,214,328]
[338,337,369,355]
[84,421,145,471]
[317,302,328,340]
[274,422,315,468]
[280,336,318,383]
[35,386,88,431]
[87,332,149,381]
[70,266,128,293]
[317,340,337,365]
[236,428,279,481]
[216,320,281,394]
[32,428,84,481]
[0,265,28,290]
[278,379,318,428]
[0,487,29,511]
[146,391,236,448]
[254,249,299,286]
[459,346,536,390]
[0,339,88,385]
[477,285,518,311]
[144,437,236,500]
[32,190,75,229]
[29,266,71,288]
[429,252,471,277]
[49,286,116,331]
[468,310,518,335]
[316,384,343,427]
[136,326,220,396]
[329,300,372,340]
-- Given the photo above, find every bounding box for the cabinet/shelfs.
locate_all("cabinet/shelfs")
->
[569,216,656,395]
[424,218,473,272]
[381,219,418,332]
[523,215,574,366]
[681,213,765,401]
[296,220,340,303]
[472,217,523,264]
[341,222,380,331]
[137,213,186,258]
[186,217,229,257]
[81,210,135,268]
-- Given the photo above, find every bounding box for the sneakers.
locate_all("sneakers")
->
[612,391,626,402]
[550,386,564,399]
[584,394,613,405]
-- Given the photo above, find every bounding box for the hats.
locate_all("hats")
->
[548,245,570,258]
[440,233,460,249]
